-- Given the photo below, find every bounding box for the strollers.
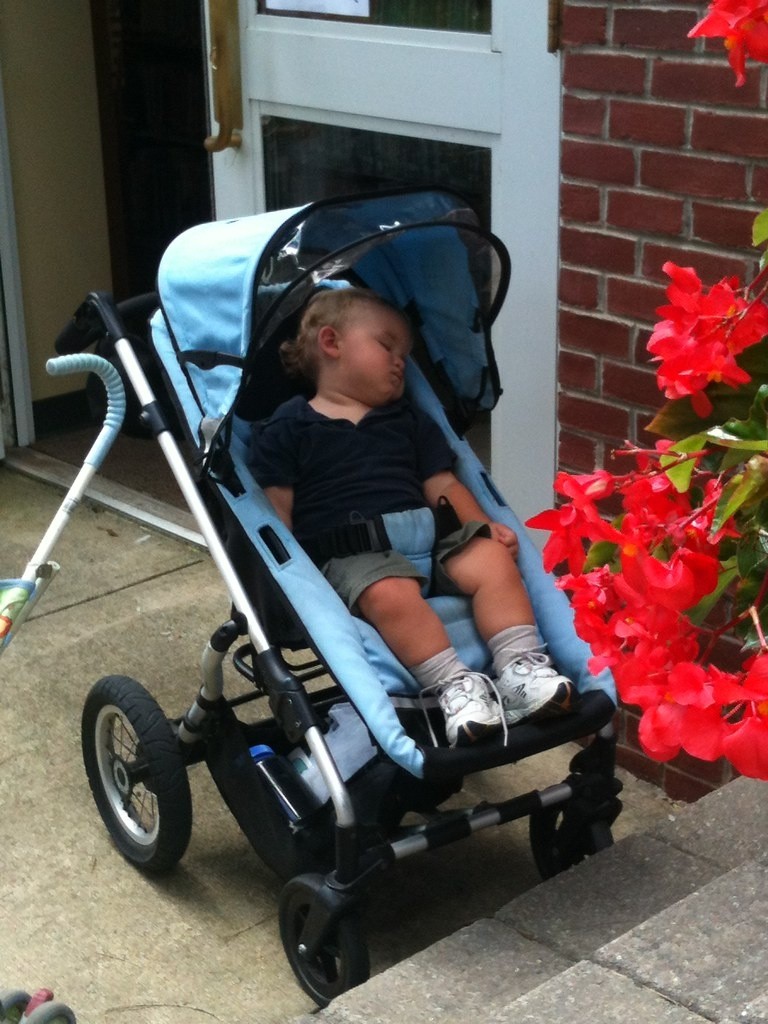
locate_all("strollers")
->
[53,183,628,1008]
[1,351,132,1024]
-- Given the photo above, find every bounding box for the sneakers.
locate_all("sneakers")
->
[419,670,509,748]
[494,642,578,727]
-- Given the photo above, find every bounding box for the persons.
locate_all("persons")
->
[255,289,579,751]
